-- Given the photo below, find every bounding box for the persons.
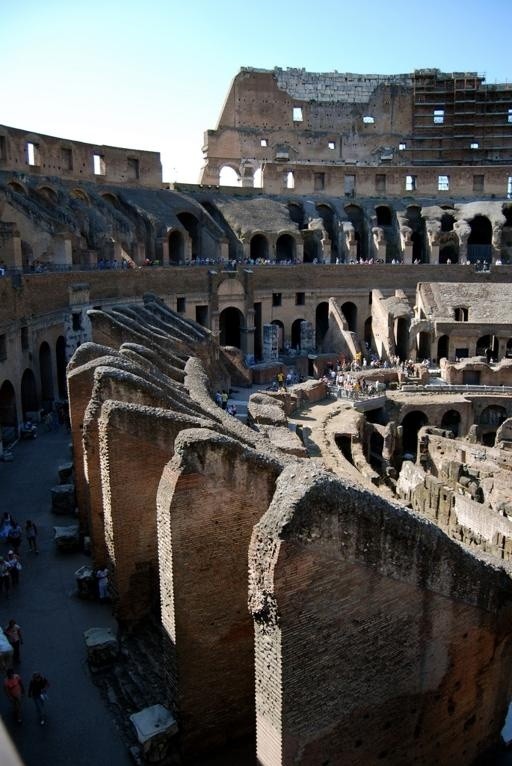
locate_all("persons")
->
[142,259,159,265]
[1,511,22,595]
[23,397,72,434]
[98,259,133,270]
[391,257,421,264]
[280,257,301,265]
[24,518,41,554]
[96,564,112,602]
[463,257,503,271]
[228,405,237,415]
[476,346,492,365]
[215,390,223,406]
[4,619,27,663]
[222,390,230,407]
[1,668,26,726]
[312,256,385,265]
[184,256,276,271]
[447,258,454,264]
[271,337,430,403]
[28,670,51,726]
